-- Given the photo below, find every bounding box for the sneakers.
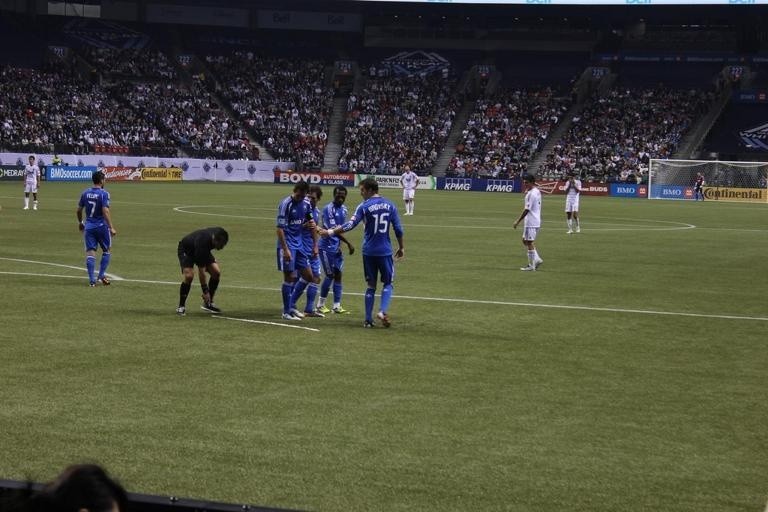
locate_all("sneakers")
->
[88,276,110,286]
[364,320,376,328]
[377,311,391,327]
[176,306,185,316]
[281,305,350,320]
[202,302,220,313]
[520,258,543,271]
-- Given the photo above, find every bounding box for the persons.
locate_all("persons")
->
[318,178,404,329]
[276,181,319,321]
[317,185,354,314]
[77,170,117,288]
[694,172,706,202]
[338,62,766,187]
[175,226,229,317]
[0,49,339,173]
[22,155,41,210]
[398,166,419,216]
[291,185,326,318]
[0,463,128,512]
[513,175,544,271]
[563,172,581,234]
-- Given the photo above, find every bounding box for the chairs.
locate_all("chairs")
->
[2,139,256,161]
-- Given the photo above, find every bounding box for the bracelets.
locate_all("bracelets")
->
[201,283,210,295]
